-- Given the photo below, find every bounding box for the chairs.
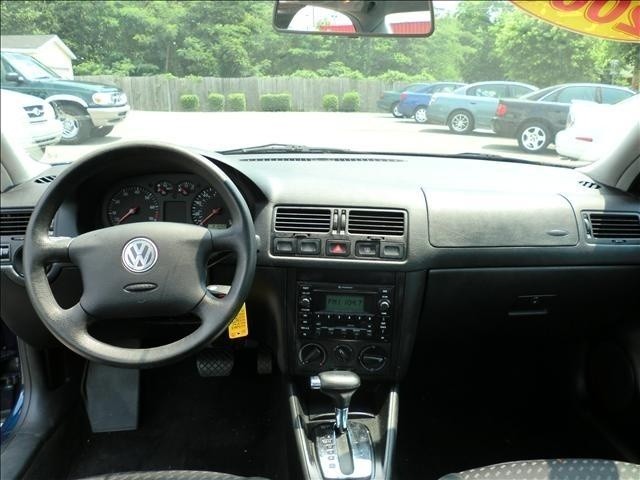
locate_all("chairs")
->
[434,457,640,480]
[79,468,275,480]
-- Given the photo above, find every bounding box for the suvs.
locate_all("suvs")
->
[1,53,129,144]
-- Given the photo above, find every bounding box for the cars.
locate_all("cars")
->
[425,82,539,136]
[397,83,469,123]
[491,81,637,154]
[377,84,414,118]
[0,90,63,157]
[554,94,639,161]
